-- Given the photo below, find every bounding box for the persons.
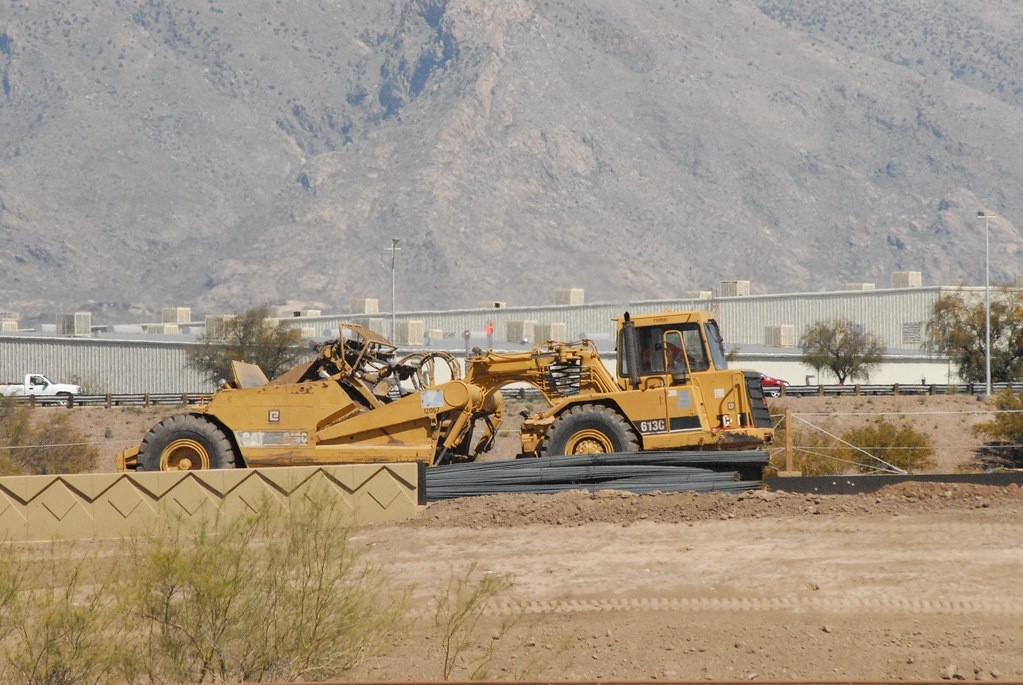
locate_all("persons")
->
[31,378,36,385]
[642,328,695,371]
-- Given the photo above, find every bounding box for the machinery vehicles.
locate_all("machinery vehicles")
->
[116,309,777,473]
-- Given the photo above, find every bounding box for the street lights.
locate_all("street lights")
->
[978,213,999,394]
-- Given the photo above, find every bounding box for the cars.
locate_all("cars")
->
[758,372,792,398]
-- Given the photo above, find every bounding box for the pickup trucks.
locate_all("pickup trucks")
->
[0,373,81,407]
